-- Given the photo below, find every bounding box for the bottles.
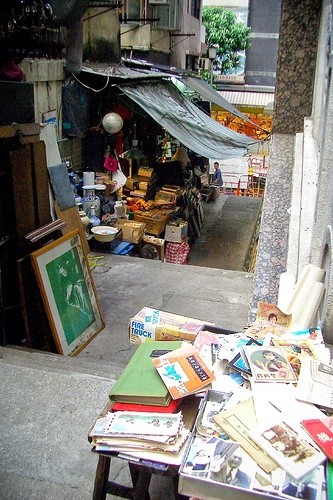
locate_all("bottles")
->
[89,207,100,226]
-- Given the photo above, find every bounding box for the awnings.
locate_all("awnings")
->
[122,55,193,77]
[80,61,272,162]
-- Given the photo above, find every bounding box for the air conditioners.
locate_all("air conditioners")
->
[194,58,208,70]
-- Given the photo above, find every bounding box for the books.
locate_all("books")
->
[152,343,216,400]
[212,326,332,480]
[109,339,183,407]
[87,395,190,472]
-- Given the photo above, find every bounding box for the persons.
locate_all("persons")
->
[212,162,224,188]
[281,469,318,500]
[210,452,251,489]
[192,449,210,476]
[255,350,288,373]
[258,313,281,346]
[308,327,320,341]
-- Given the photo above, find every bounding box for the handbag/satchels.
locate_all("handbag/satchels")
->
[165,241,190,263]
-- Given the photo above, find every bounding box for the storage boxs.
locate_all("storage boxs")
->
[95,166,180,262]
[190,324,239,347]
[197,173,208,187]
[176,388,326,500]
[128,306,215,346]
[165,220,188,244]
[204,184,220,202]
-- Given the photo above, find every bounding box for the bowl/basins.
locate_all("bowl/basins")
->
[91,225,118,242]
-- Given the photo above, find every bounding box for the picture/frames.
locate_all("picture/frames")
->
[16,254,49,354]
[30,227,106,358]
[22,217,68,354]
[181,186,205,247]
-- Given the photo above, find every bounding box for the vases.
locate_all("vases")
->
[69,174,77,197]
[89,207,101,227]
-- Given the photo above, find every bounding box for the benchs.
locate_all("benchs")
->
[131,395,202,500]
[89,401,151,500]
